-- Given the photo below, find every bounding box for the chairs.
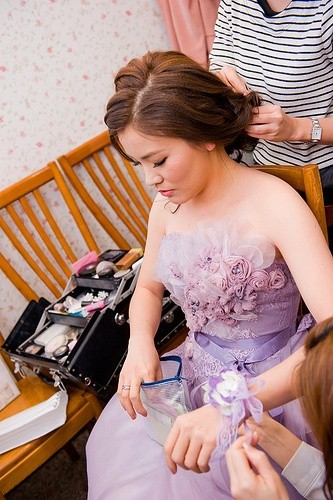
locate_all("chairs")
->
[0,160,102,304]
[56,128,157,251]
[0,334,104,500]
[243,163,329,250]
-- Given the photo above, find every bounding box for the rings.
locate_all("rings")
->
[122,385,130,391]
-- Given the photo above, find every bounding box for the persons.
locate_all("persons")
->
[209,0,333,206]
[85,50,333,500]
[224,316,333,500]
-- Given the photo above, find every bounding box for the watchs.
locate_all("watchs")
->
[306,118,322,144]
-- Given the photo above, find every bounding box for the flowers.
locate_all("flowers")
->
[200,368,267,462]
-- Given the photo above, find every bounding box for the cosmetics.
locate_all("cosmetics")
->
[20,246,143,364]
